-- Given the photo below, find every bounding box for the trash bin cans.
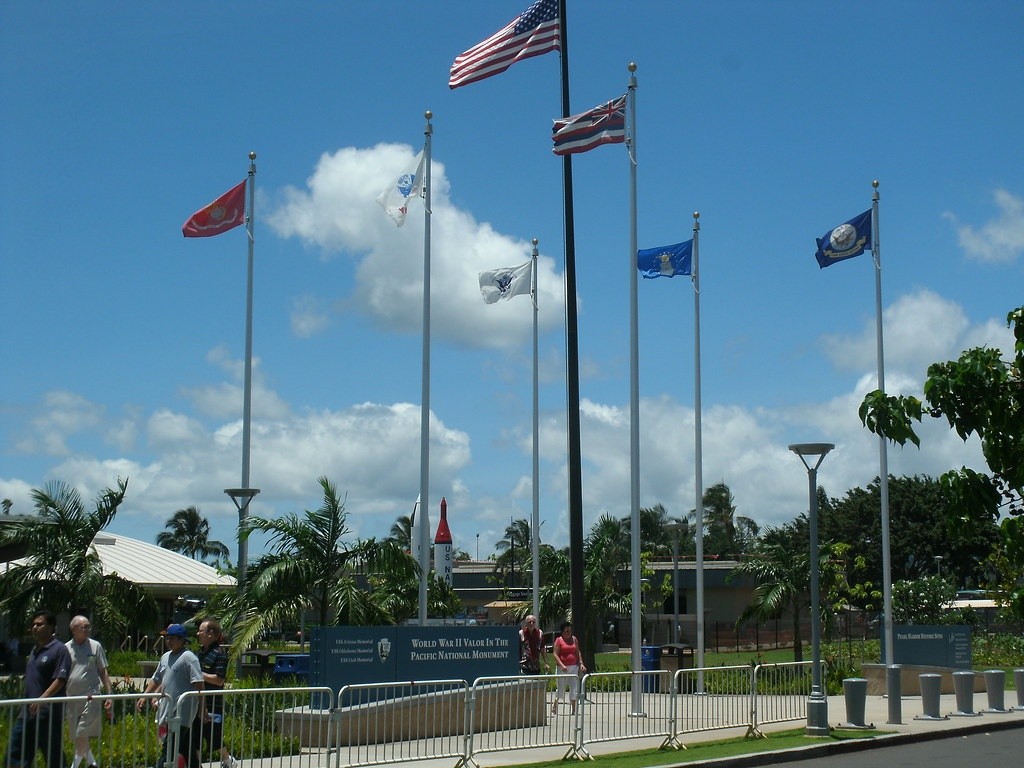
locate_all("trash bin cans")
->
[659,643,694,694]
[274,653,310,684]
[630,646,659,691]
[240,650,279,683]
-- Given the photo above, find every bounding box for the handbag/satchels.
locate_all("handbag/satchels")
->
[575,638,580,665]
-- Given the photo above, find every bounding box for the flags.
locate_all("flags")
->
[181,180,245,238]
[816,208,872,270]
[637,239,692,279]
[376,149,425,228]
[478,260,531,304]
[449,0,561,91]
[552,95,625,156]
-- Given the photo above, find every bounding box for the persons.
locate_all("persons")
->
[137,624,209,768]
[3,612,72,768]
[606,620,614,639]
[63,615,113,768]
[518,614,550,675]
[552,622,586,715]
[196,620,238,768]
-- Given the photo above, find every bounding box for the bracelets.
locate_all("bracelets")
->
[580,663,582,665]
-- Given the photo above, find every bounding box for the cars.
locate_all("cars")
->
[271,625,302,644]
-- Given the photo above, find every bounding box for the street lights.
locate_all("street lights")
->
[662,519,691,646]
[788,443,838,738]
[934,555,943,577]
[223,487,262,608]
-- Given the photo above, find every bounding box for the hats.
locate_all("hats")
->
[167,624,187,636]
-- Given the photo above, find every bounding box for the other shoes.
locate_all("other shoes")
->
[552,704,556,714]
[572,711,580,715]
[222,757,239,768]
